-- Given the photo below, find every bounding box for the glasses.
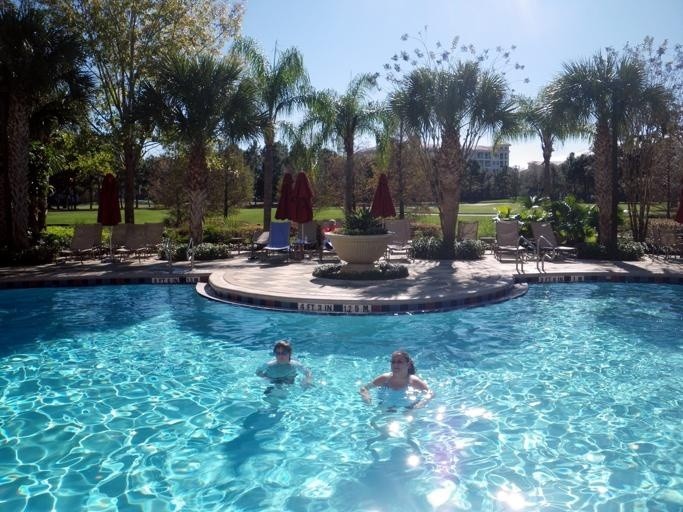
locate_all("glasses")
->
[274,351,288,355]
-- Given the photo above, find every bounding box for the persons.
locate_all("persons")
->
[256,339,311,390]
[356,348,434,417]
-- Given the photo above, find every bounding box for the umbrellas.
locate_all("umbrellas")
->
[273,169,295,222]
[288,169,316,243]
[369,172,397,260]
[95,170,122,257]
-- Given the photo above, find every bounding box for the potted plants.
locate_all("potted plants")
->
[324,203,397,274]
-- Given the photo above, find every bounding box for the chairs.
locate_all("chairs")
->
[458,217,577,262]
[653,223,683,262]
[247,220,343,264]
[58,220,166,266]
[384,218,416,263]
